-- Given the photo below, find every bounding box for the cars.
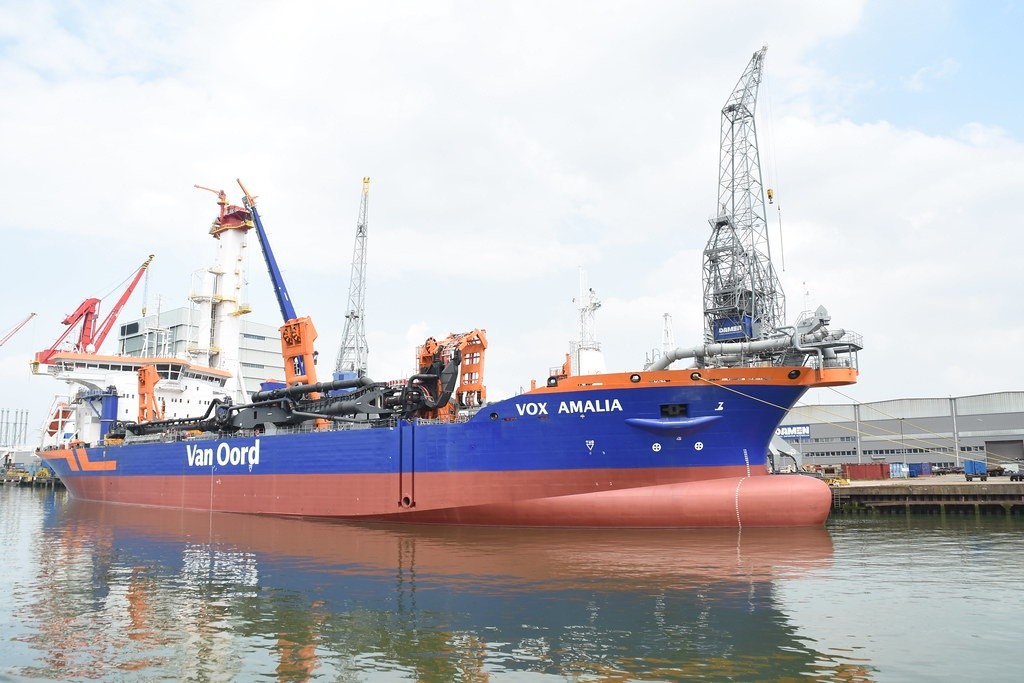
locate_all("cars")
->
[932,466,965,472]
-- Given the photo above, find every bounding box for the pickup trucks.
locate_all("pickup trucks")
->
[1010,470,1024,481]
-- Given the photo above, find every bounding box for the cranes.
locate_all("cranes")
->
[697,39,795,366]
[333,176,375,394]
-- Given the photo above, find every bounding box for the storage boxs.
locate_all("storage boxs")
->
[841,461,932,480]
[964,458,987,475]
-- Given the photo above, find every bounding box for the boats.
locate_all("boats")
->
[31,305,863,533]
